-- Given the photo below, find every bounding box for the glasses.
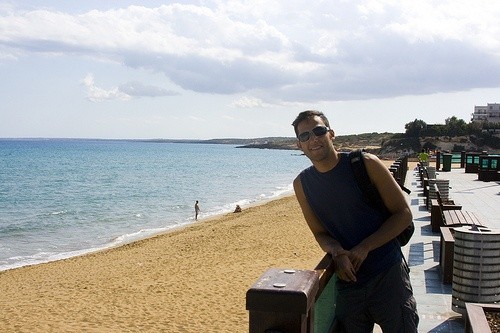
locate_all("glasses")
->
[297,125,331,143]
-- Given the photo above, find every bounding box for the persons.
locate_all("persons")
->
[234,204,241,213]
[418,149,429,167]
[292,110,419,333]
[195,200,200,219]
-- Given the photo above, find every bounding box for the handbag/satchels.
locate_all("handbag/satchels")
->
[350,148,415,248]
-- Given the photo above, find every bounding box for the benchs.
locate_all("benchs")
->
[424,167,430,179]
[442,209,488,229]
[434,183,461,210]
